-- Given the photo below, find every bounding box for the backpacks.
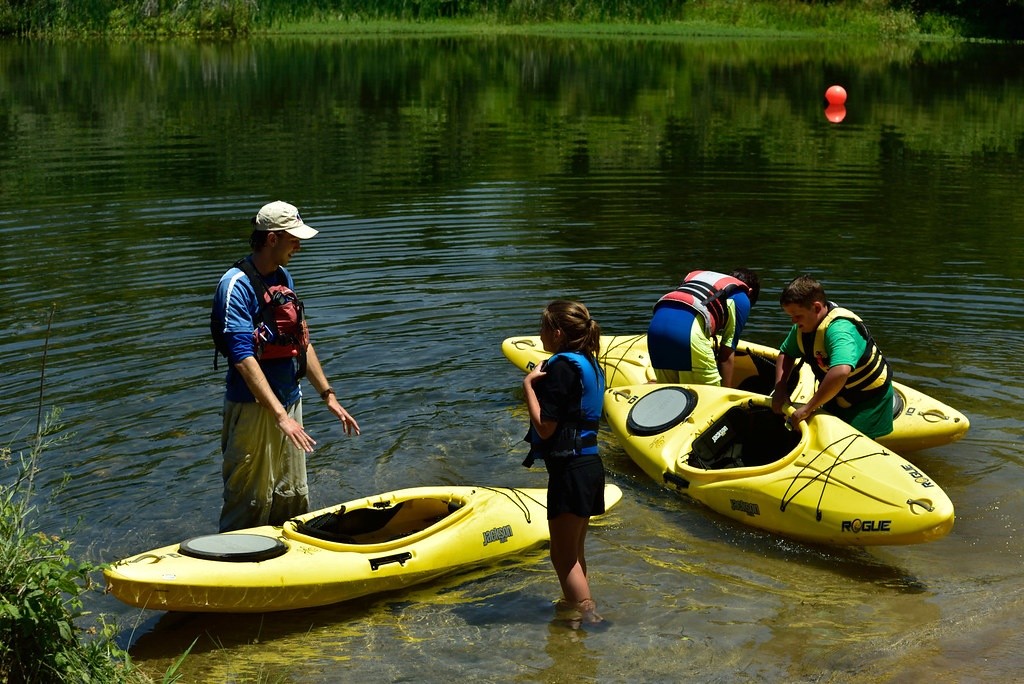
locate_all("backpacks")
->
[255,286,308,363]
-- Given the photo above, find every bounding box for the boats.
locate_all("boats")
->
[99,480,621,616]
[498,332,971,453]
[600,375,957,551]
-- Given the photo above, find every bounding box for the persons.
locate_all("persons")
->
[522,297,607,603]
[210,200,361,535]
[645,268,757,390]
[772,274,894,440]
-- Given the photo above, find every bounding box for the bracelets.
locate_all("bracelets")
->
[320,387,336,401]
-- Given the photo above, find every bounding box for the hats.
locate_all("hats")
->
[253,200,319,241]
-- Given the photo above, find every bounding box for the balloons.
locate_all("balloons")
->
[824,104,847,123]
[826,86,846,104]
